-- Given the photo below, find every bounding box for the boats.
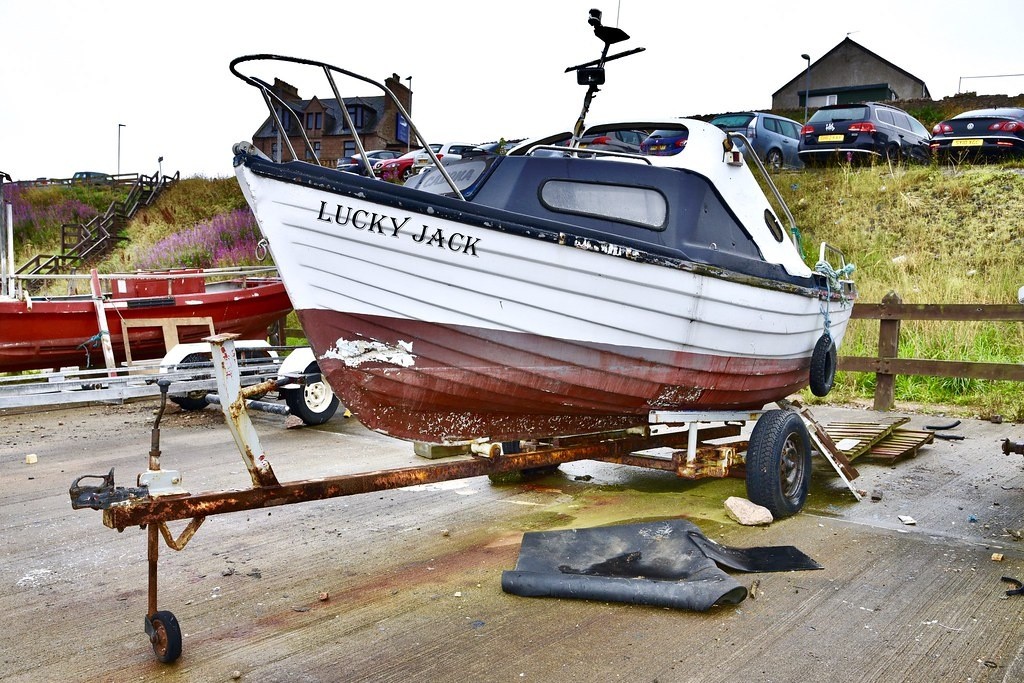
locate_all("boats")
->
[0,203,295,373]
[226,0,859,440]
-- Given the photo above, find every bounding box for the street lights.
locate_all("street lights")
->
[404,76,413,153]
[801,53,811,125]
[117,124,126,185]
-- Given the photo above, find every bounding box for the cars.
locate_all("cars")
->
[373,149,421,182]
[639,130,689,156]
[33,177,69,190]
[929,105,1024,166]
[336,149,405,176]
[472,138,567,157]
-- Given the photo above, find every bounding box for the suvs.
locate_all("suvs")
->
[70,170,116,188]
[796,100,933,168]
[411,142,492,177]
[707,111,805,174]
[565,129,650,159]
